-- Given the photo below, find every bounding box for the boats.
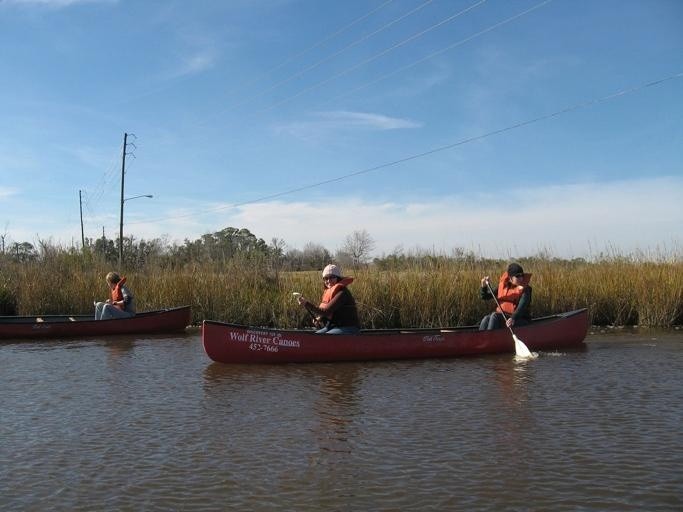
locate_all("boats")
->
[0,301,192,342]
[201,304,588,366]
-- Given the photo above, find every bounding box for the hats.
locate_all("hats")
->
[508,263,523,276]
[322,264,340,277]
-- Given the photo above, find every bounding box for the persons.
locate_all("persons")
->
[93,272,135,321]
[479,262,533,332]
[296,263,362,336]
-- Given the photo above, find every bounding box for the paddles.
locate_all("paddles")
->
[486,281,535,361]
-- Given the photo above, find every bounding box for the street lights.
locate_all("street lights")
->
[119,194,153,271]
